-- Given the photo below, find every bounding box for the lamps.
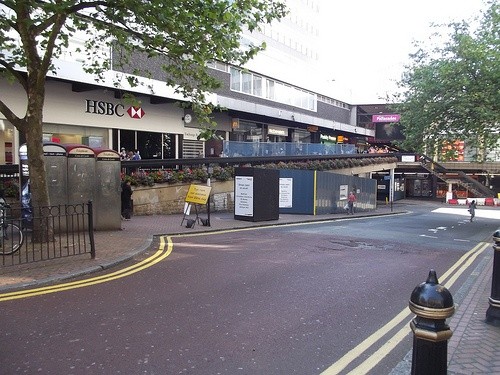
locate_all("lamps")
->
[182,114,192,123]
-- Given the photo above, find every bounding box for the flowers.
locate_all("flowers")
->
[122,156,399,187]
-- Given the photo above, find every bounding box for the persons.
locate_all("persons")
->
[347,192,355,215]
[121,179,134,221]
[469,200,477,222]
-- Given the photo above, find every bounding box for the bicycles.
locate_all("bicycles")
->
[0,197,24,255]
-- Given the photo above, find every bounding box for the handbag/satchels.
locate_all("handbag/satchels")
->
[344,204,349,210]
